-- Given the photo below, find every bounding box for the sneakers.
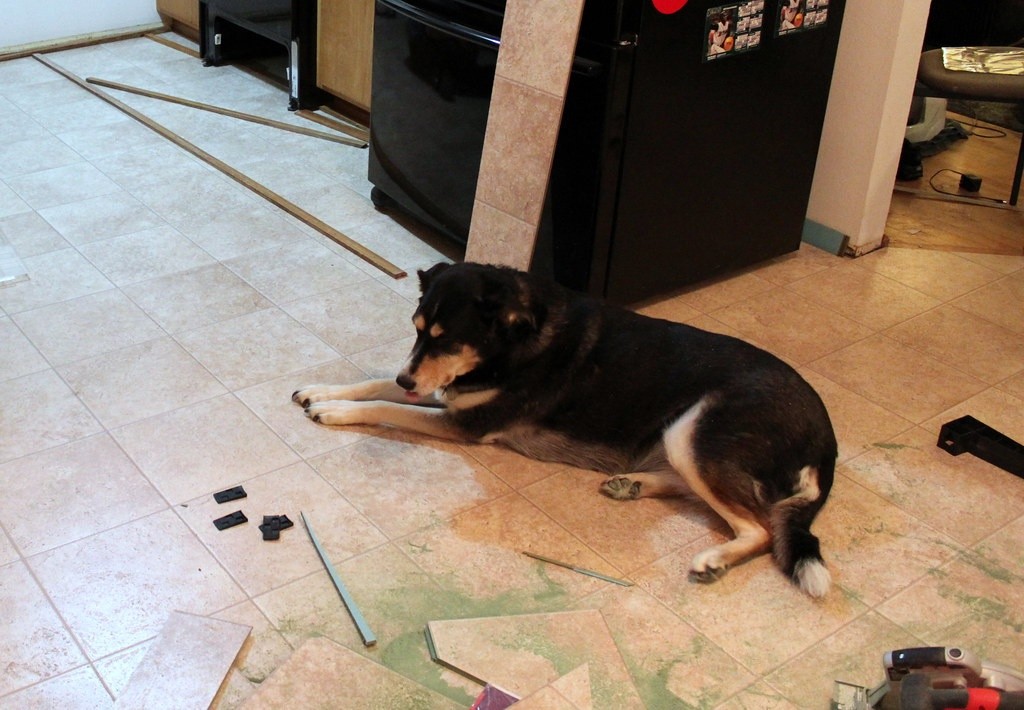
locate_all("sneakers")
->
[896,136,924,181]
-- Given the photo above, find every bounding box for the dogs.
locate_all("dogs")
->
[292,263,838,598]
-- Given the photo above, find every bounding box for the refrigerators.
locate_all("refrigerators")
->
[362,1,849,307]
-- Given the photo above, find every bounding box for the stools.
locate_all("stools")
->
[916,44,1024,207]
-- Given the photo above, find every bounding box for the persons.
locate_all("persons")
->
[708,11,729,47]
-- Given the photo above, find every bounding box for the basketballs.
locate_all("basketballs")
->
[723,37,734,51]
[794,13,803,27]
